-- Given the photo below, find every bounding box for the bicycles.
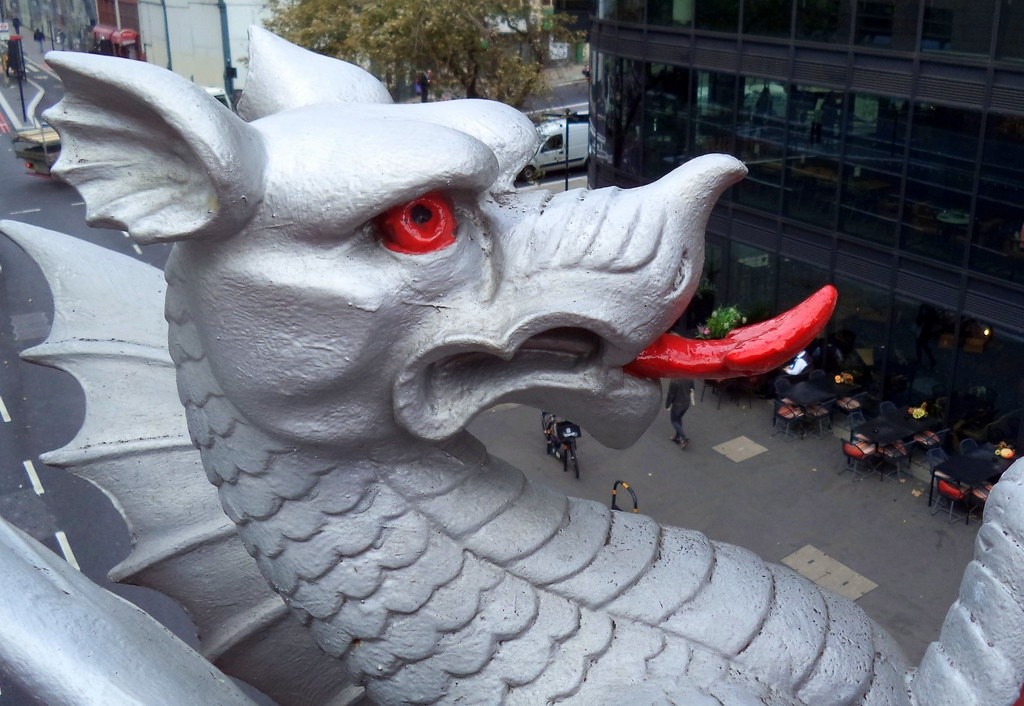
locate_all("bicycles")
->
[542,411,579,477]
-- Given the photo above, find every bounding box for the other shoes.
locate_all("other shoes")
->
[681,437,689,449]
[670,436,679,441]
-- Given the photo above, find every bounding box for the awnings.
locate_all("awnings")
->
[93,24,113,40]
[111,29,138,45]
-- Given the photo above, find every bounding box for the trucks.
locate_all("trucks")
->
[517,111,589,181]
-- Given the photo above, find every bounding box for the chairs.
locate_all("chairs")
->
[700,370,997,523]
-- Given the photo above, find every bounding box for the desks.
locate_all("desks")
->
[771,379,837,440]
[965,441,1021,474]
[928,454,997,525]
[846,416,915,483]
[813,371,864,424]
[886,403,940,466]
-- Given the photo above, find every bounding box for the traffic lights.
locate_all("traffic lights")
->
[7,41,22,70]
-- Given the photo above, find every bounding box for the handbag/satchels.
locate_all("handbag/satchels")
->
[690,389,695,406]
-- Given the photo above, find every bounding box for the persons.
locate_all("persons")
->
[665,378,695,448]
[13,17,20,34]
[59,32,66,50]
[421,71,430,102]
[34,29,46,52]
